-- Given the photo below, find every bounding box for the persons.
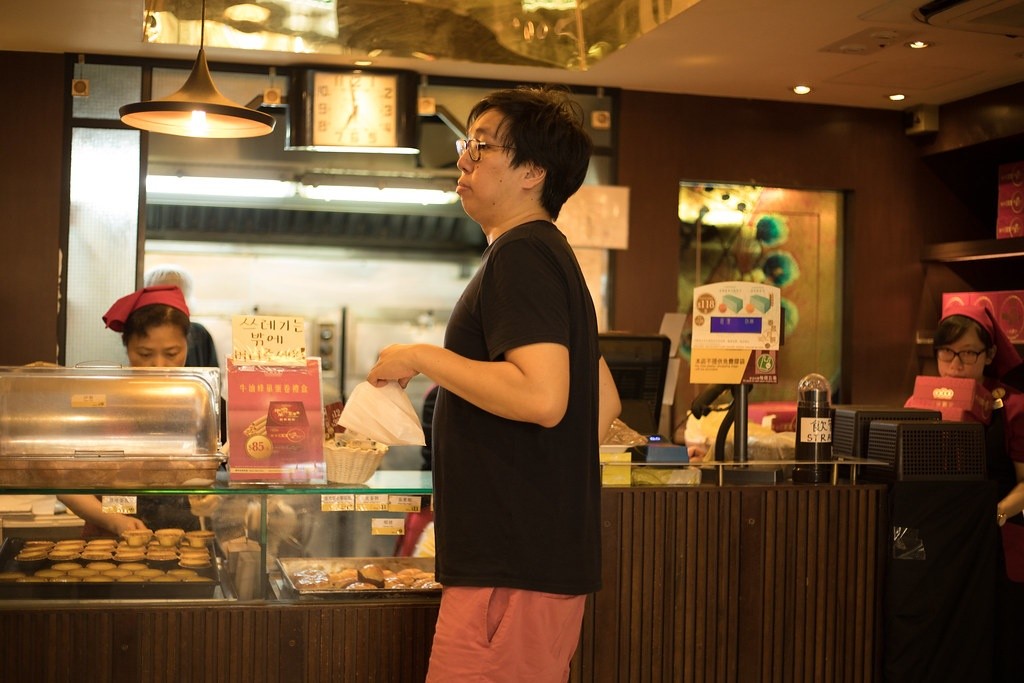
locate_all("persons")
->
[56,284,229,542]
[367,89,621,683]
[395,385,439,557]
[144,264,218,367]
[881,304,1024,683]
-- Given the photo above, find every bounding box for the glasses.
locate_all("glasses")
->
[935,348,986,364]
[455,139,517,162]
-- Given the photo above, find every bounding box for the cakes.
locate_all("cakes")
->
[293,566,442,590]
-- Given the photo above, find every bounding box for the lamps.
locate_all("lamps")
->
[118,1,277,141]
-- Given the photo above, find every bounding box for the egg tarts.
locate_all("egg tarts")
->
[0,529,215,582]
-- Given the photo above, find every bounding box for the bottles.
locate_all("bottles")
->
[792,388,832,485]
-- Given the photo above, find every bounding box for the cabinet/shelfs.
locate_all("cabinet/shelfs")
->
[902,239,1024,408]
[1,472,442,683]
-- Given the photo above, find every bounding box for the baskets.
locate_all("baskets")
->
[323,436,388,484]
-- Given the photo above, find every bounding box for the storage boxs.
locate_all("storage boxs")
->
[832,403,991,483]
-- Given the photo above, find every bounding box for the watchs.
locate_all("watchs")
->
[997,512,1007,527]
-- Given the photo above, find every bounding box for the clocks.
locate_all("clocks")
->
[287,63,420,154]
